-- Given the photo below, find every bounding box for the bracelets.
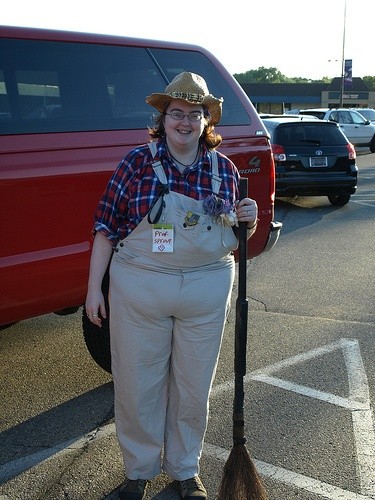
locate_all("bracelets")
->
[247,224,256,229]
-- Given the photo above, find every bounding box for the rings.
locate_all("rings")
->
[92,315,97,317]
[247,211,249,215]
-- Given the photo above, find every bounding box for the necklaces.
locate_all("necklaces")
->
[166,142,199,168]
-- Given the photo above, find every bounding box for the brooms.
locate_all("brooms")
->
[217,177,265,500]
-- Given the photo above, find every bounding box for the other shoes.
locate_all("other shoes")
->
[120,478,149,500]
[181,476,207,500]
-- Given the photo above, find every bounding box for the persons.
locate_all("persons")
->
[85,72,258,500]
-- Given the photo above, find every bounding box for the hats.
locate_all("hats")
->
[145,71,224,124]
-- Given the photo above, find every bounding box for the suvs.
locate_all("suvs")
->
[299,108,375,153]
[259,113,359,208]
[0,25,283,334]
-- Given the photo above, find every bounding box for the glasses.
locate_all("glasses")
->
[165,111,203,122]
